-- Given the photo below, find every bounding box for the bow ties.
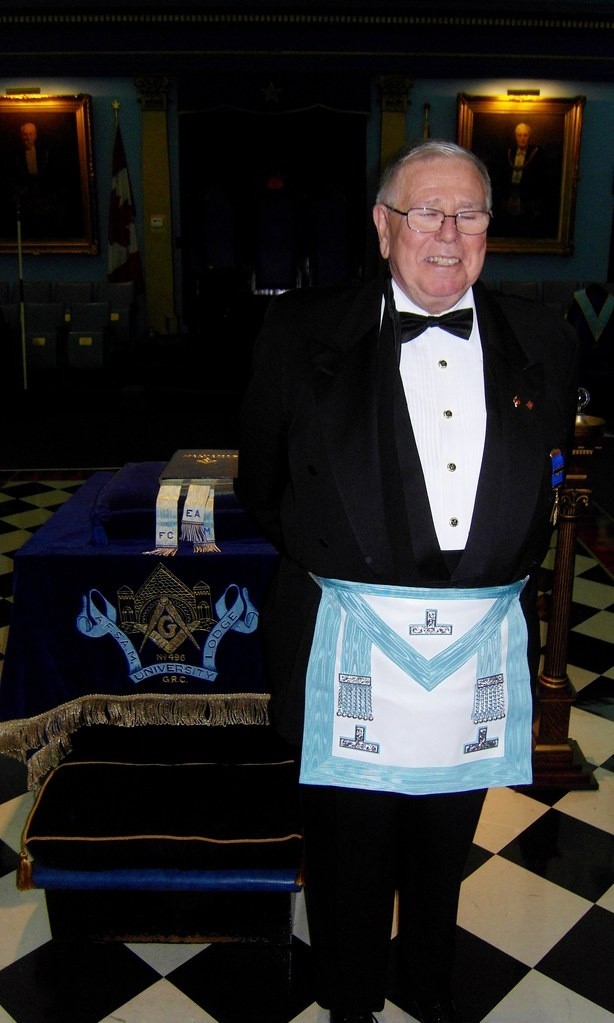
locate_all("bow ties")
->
[392,307,473,343]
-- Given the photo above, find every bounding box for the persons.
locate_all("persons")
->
[239,139,577,1023]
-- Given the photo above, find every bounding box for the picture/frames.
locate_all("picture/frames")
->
[0,94,100,256]
[458,92,585,257]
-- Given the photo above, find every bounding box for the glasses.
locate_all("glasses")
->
[385,204,491,235]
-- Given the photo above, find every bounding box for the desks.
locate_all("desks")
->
[1,459,283,791]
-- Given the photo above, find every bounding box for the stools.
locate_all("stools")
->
[18,759,302,944]
